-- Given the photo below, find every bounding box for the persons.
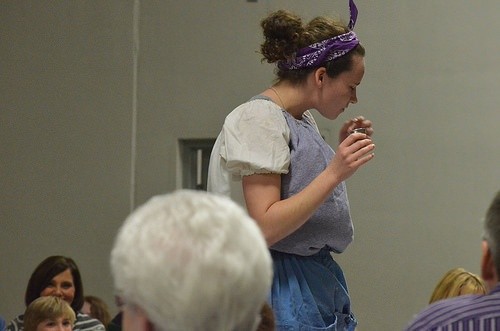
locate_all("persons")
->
[400,191,500,331]
[0,188,279,331]
[208,0,375,331]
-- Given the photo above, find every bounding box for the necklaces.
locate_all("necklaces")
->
[269,85,286,109]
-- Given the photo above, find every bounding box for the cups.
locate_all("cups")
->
[346,127,366,152]
